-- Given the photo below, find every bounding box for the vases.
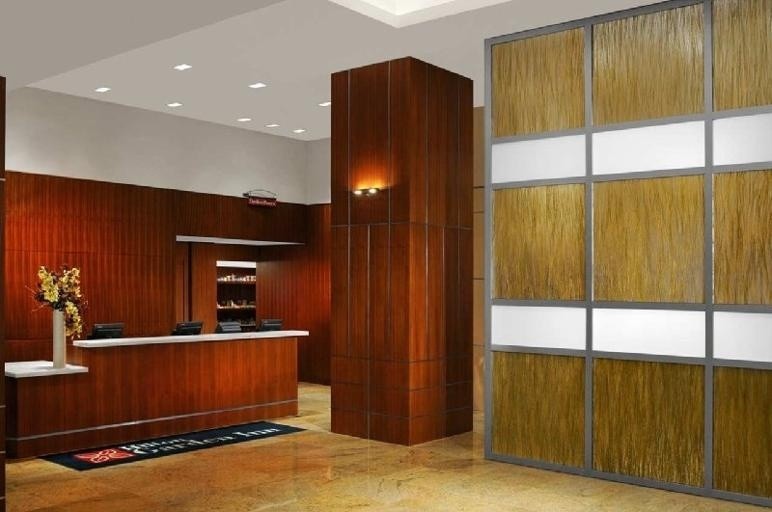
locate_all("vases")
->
[52,306,68,368]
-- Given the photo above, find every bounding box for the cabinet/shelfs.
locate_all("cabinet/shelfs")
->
[217,278,256,327]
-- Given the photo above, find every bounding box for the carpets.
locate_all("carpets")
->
[36,421,307,472]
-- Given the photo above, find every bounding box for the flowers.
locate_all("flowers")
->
[28,266,87,340]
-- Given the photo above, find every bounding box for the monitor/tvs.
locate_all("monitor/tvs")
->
[92,323,124,340]
[175,322,203,335]
[258,319,283,331]
[215,321,242,333]
